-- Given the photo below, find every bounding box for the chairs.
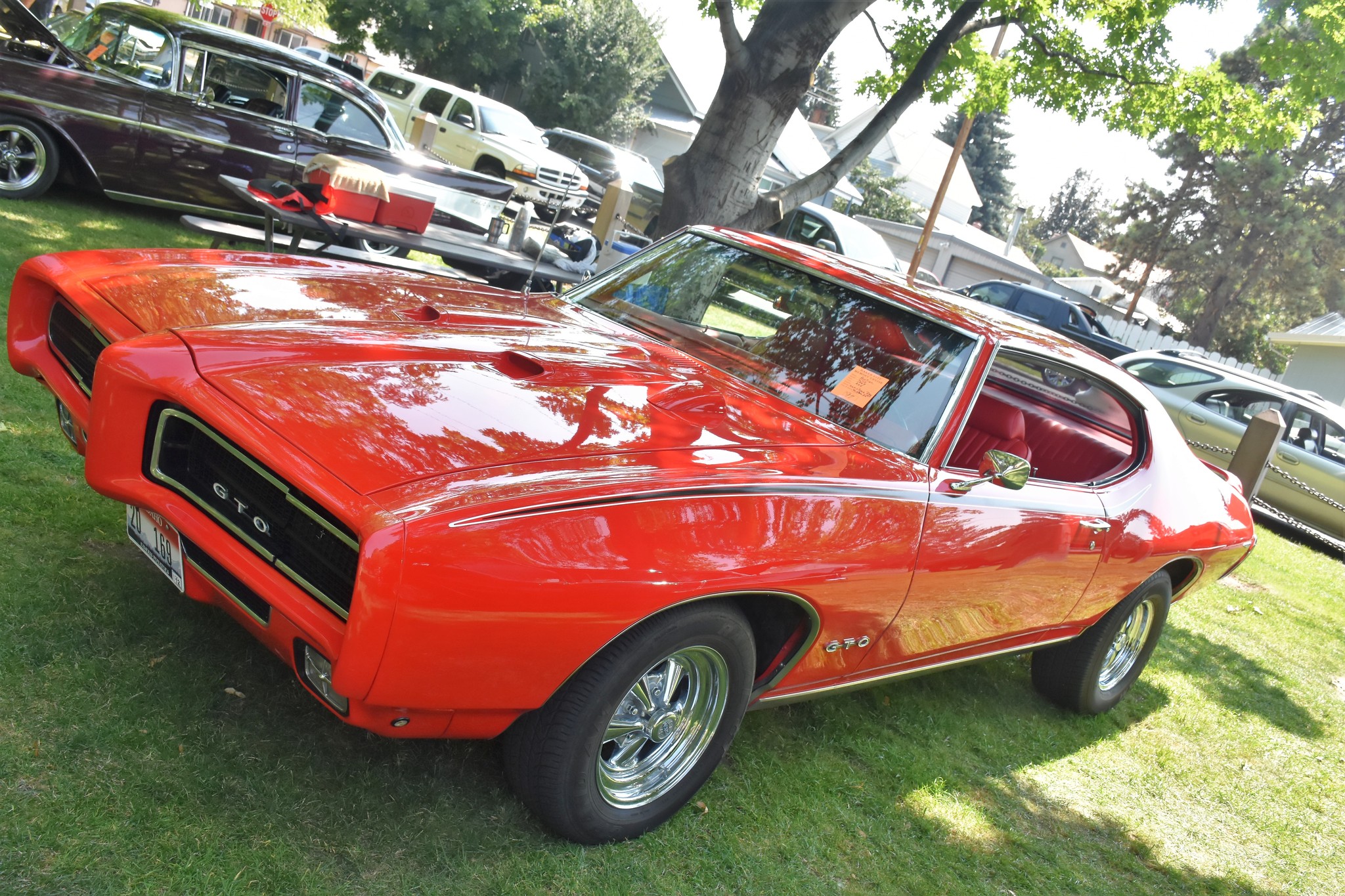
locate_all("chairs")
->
[764,316,1034,475]
[1292,427,1320,455]
[212,82,232,104]
[243,98,283,117]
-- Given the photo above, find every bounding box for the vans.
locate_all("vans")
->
[290,48,365,86]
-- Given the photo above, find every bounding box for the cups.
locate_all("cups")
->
[487,217,510,244]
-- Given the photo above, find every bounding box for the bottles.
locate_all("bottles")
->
[507,201,534,253]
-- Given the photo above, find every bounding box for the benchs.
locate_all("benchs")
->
[181,214,489,286]
[1204,398,1235,420]
[842,309,1128,483]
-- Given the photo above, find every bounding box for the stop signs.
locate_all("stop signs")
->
[260,3,278,22]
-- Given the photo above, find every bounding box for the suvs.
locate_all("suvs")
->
[541,127,665,241]
[1078,347,1345,552]
[350,66,590,224]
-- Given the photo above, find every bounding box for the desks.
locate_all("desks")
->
[217,174,593,294]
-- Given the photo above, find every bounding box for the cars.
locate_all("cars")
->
[4,223,1260,844]
[0,1,515,261]
[925,279,1144,392]
[86,1,167,62]
[754,188,908,267]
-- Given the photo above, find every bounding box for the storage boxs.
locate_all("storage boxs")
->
[309,153,380,223]
[595,230,655,285]
[376,175,437,234]
